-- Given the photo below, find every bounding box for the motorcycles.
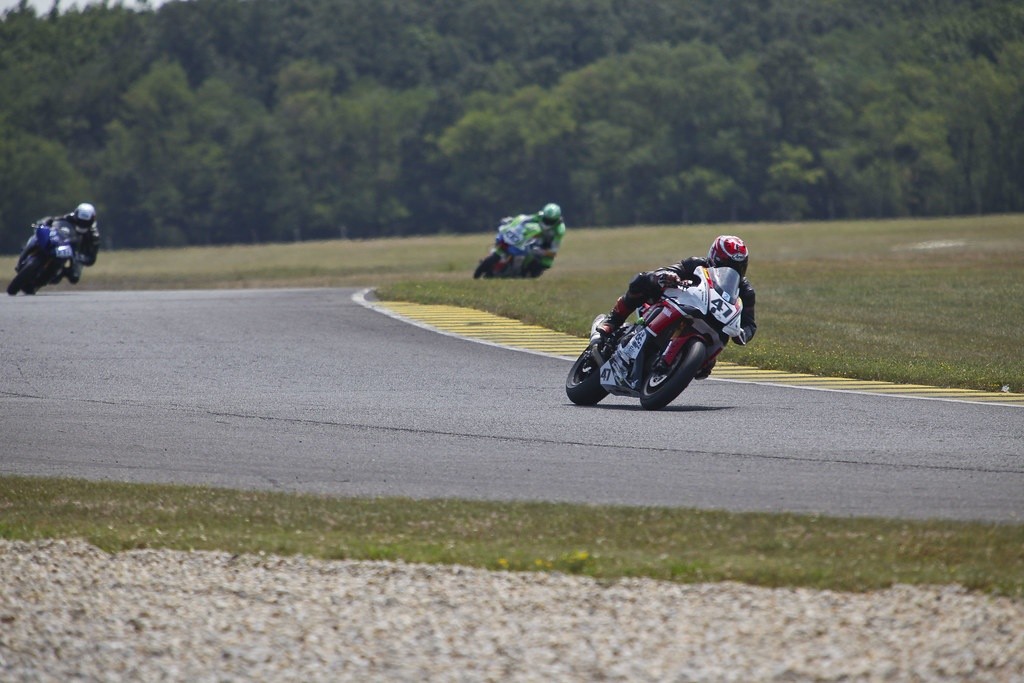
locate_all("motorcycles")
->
[473,215,545,282]
[565,264,746,411]
[7,220,83,295]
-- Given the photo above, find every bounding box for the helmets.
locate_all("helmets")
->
[73,203,95,234]
[540,203,561,229]
[708,234,749,278]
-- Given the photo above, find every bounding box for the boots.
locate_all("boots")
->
[597,296,634,335]
[695,345,722,378]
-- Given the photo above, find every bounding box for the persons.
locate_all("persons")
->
[15,203,101,285]
[495,203,567,275]
[596,234,758,382]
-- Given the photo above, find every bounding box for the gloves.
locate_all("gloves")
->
[36,217,52,226]
[532,246,545,257]
[71,251,88,264]
[500,216,513,225]
[652,270,680,290]
[731,326,753,346]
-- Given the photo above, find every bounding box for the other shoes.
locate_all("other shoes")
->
[50,266,65,284]
[15,257,24,272]
[523,265,540,279]
